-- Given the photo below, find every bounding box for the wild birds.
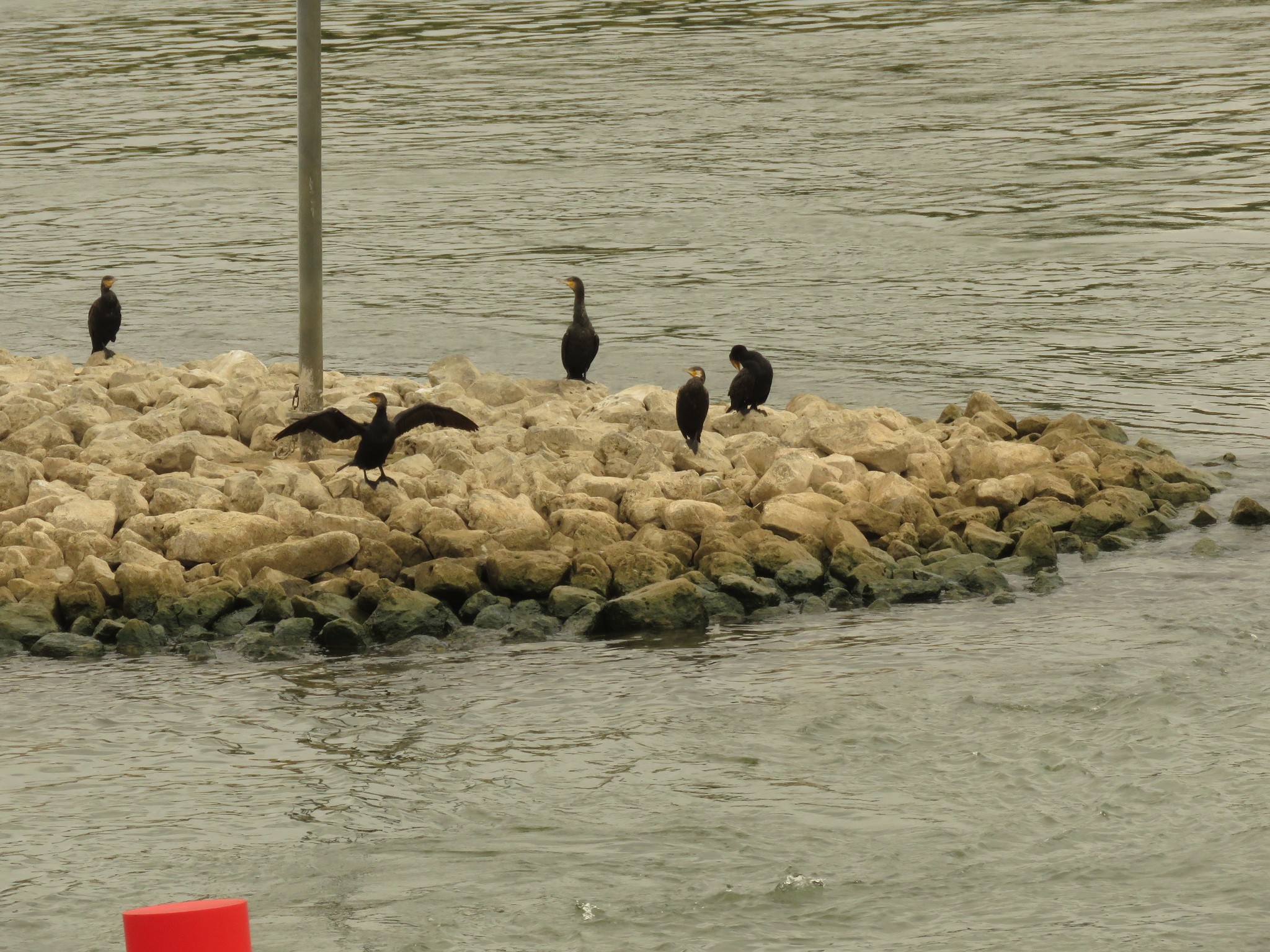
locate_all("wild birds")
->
[554,274,601,386]
[725,344,774,419]
[674,365,710,457]
[87,275,122,360]
[270,391,480,491]
